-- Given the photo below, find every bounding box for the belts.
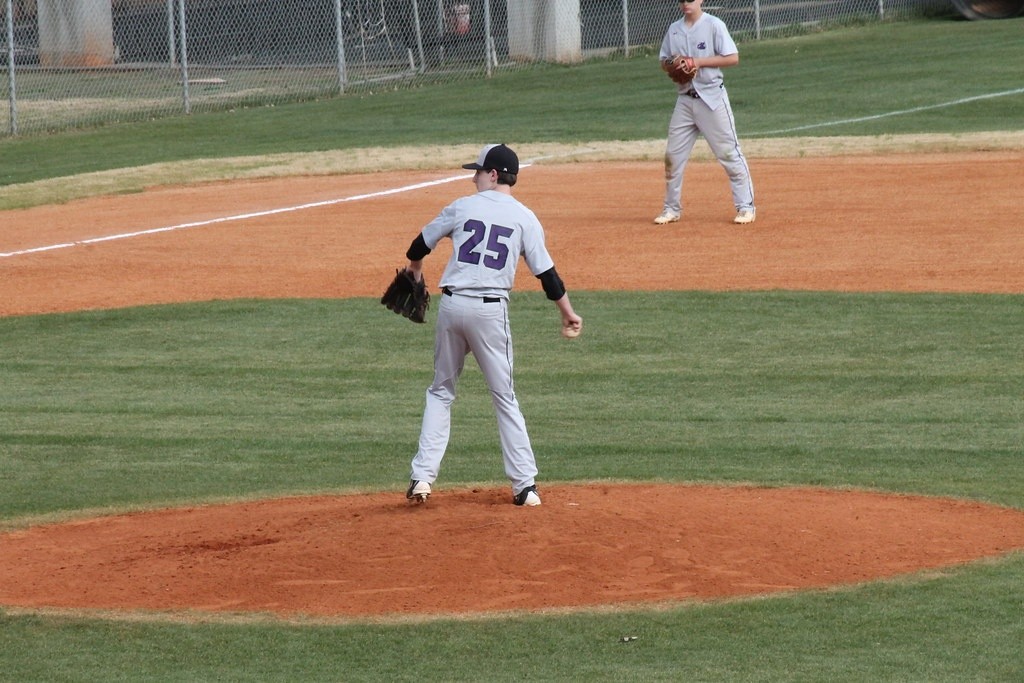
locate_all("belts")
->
[443,287,501,303]
[686,83,722,99]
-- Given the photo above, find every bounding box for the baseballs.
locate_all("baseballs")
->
[566,326,579,338]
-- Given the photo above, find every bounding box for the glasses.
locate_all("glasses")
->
[679,0,695,2]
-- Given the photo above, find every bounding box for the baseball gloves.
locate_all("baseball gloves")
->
[380,265,432,325]
[663,53,698,86]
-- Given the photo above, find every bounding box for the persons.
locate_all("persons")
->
[405,145,582,507]
[654,0,756,224]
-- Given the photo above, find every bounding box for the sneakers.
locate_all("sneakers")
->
[734,208,755,225]
[406,479,431,503]
[514,486,541,506]
[654,207,680,224]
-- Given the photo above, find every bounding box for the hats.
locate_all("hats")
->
[461,143,519,174]
